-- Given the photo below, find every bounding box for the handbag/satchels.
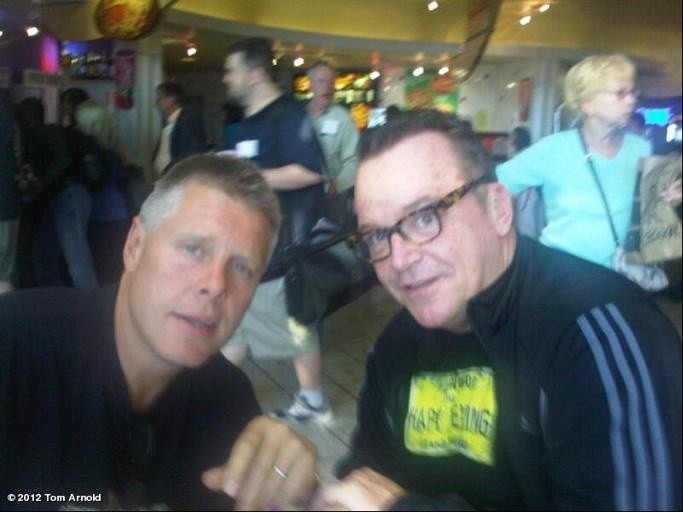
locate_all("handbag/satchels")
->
[278,231,379,323]
[613,244,670,294]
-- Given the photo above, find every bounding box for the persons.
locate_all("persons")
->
[0,36,682,511]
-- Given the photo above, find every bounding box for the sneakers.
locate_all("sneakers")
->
[265,390,334,427]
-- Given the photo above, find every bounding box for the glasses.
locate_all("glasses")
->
[596,86,642,100]
[343,171,500,266]
